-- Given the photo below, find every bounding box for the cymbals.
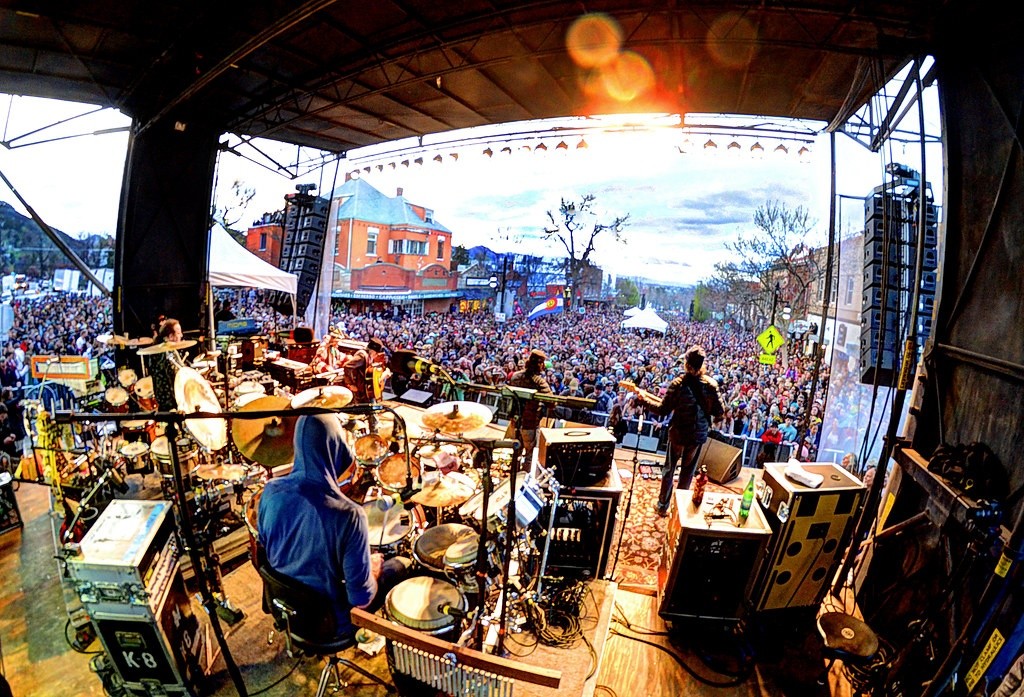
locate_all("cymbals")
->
[290,386,353,409]
[195,350,221,363]
[174,365,228,452]
[409,470,475,507]
[192,361,217,369]
[231,394,300,468]
[96,331,198,356]
[422,400,494,436]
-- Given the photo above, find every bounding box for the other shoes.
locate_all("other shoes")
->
[653,502,667,517]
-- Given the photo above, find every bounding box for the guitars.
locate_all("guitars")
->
[618,379,664,403]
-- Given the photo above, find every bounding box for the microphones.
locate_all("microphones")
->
[378,486,422,511]
[437,604,477,620]
[105,464,130,495]
[507,577,534,608]
[192,336,212,342]
[389,417,401,454]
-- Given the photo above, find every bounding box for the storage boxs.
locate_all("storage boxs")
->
[64,499,206,697]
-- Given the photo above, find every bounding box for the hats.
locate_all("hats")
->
[679,345,706,367]
[367,337,384,353]
[529,349,546,362]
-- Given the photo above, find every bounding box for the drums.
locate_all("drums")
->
[56,325,563,644]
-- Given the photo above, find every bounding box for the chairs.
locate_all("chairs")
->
[260,564,396,697]
[801,613,898,697]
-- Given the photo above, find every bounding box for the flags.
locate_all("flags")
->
[528,293,564,325]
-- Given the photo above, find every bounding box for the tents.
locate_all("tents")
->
[623,306,642,317]
[620,308,668,339]
[206,223,297,389]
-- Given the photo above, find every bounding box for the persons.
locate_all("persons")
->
[149,319,191,413]
[839,452,892,565]
[298,304,585,421]
[571,305,903,469]
[257,413,417,645]
[0,271,114,474]
[344,338,383,434]
[510,349,557,473]
[638,344,725,516]
[212,286,306,336]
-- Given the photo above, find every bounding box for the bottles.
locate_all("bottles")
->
[739,474,755,518]
[692,465,708,504]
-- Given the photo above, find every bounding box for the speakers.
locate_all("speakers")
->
[400,389,433,409]
[528,449,623,581]
[621,432,659,454]
[268,194,330,316]
[485,404,499,425]
[859,196,939,390]
[695,437,743,483]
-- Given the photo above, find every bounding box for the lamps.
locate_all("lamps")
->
[882,162,922,185]
[294,181,318,197]
